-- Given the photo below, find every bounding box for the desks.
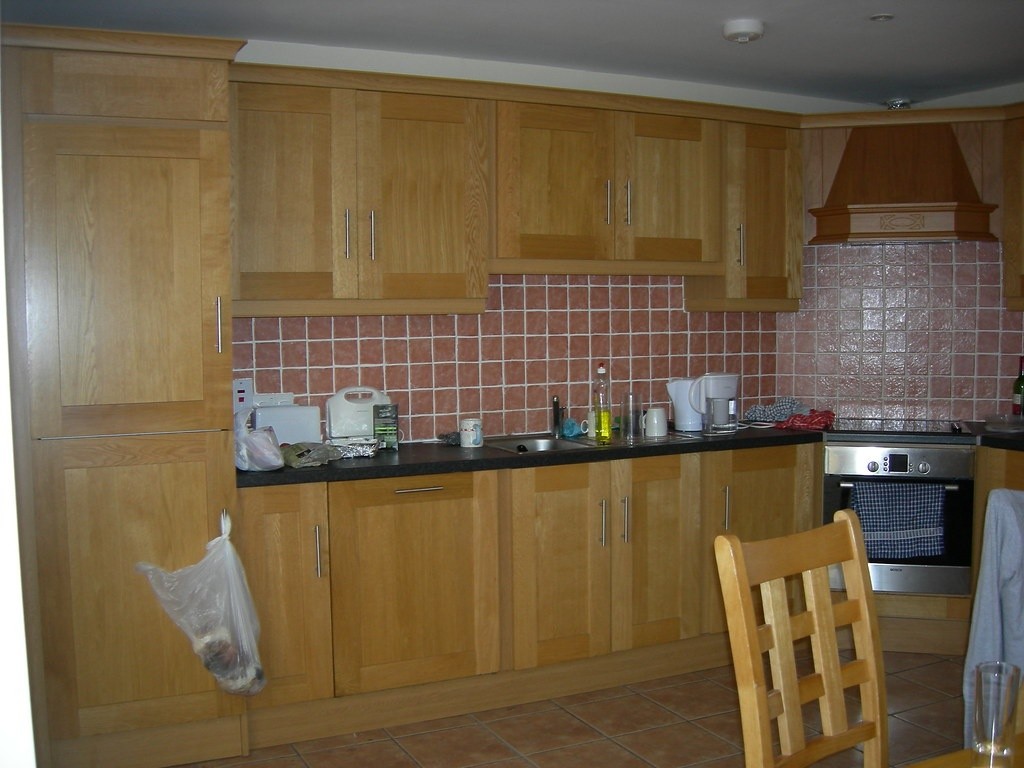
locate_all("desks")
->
[900,732,1024,768]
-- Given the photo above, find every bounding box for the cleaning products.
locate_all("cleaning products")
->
[593,362,613,446]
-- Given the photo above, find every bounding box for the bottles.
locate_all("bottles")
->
[594,363,613,446]
[1012,355,1024,416]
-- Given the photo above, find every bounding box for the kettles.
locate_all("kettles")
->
[665,377,705,432]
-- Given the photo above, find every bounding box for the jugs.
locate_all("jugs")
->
[688,372,740,435]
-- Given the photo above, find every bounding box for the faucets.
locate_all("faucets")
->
[552,395,562,440]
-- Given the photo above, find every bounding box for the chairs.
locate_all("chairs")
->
[713,507,891,768]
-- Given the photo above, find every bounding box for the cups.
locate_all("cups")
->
[459,417,484,448]
[581,411,598,437]
[971,661,1021,768]
[641,406,668,437]
[620,393,644,440]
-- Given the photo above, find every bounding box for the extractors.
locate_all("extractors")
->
[806,121,999,246]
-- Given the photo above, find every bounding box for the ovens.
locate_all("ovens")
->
[818,442,978,597]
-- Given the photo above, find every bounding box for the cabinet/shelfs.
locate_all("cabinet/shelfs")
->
[682,106,803,313]
[488,80,725,275]
[236,480,335,754]
[0,22,248,768]
[327,468,506,736]
[503,452,704,709]
[227,60,491,315]
[703,441,824,669]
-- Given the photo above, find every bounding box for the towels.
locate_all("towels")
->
[962,486,1024,749]
[848,481,947,560]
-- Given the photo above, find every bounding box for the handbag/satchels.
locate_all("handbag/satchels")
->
[135,513,265,696]
[235,408,284,471]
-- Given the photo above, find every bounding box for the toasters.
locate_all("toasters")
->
[325,386,392,442]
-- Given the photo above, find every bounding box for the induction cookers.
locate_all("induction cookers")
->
[819,414,982,449]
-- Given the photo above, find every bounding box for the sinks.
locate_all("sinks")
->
[483,434,595,455]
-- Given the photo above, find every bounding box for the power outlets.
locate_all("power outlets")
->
[252,393,293,407]
